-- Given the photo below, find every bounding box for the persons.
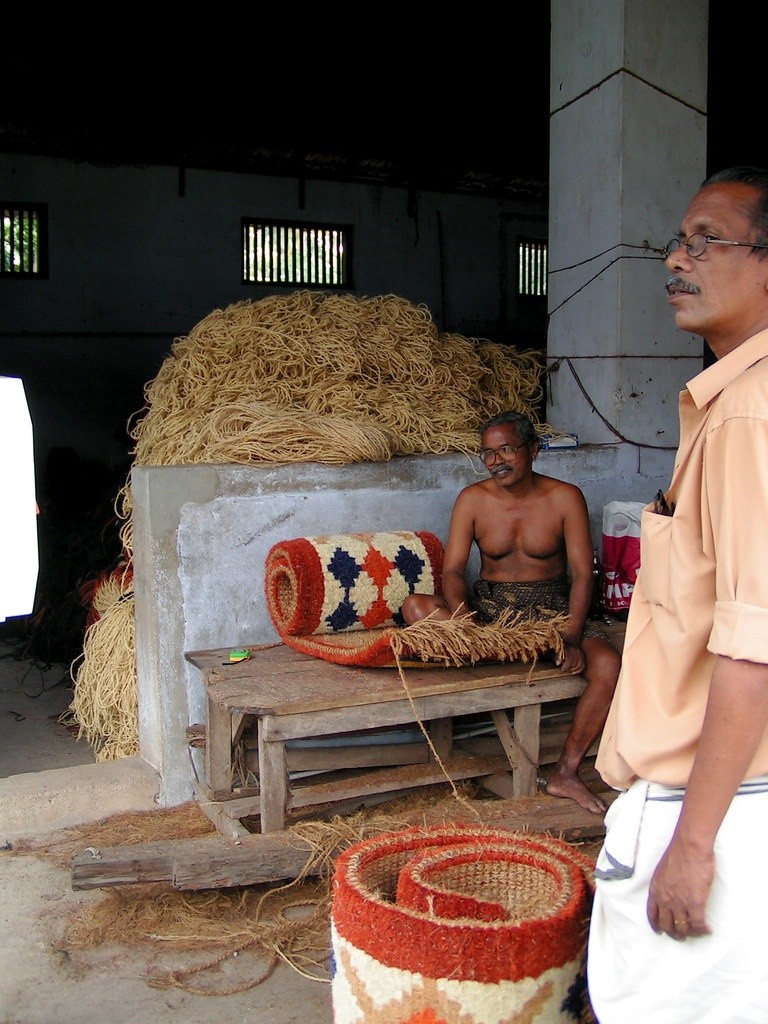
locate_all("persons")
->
[402,411,623,813]
[584,156,768,1024]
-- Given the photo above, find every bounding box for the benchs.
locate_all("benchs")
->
[183,611,629,842]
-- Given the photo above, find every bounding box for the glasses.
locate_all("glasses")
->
[667,233,768,257]
[479,440,529,466]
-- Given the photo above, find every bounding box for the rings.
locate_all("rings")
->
[675,917,690,925]
